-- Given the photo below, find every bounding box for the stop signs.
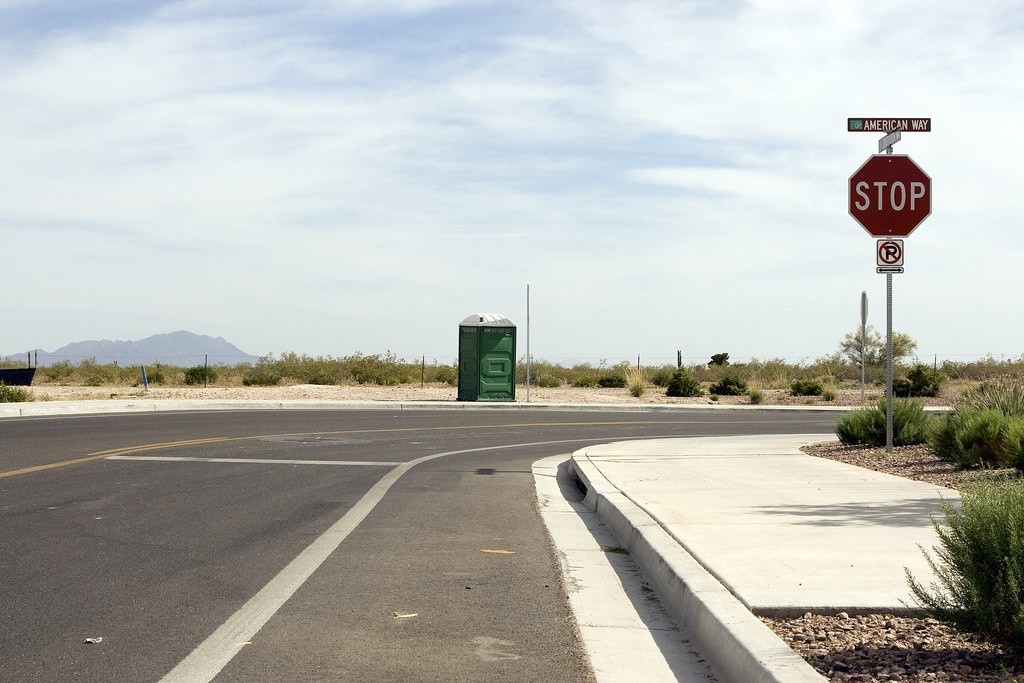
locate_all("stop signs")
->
[848,154,933,237]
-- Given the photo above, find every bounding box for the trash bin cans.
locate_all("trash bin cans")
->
[458,314,518,404]
